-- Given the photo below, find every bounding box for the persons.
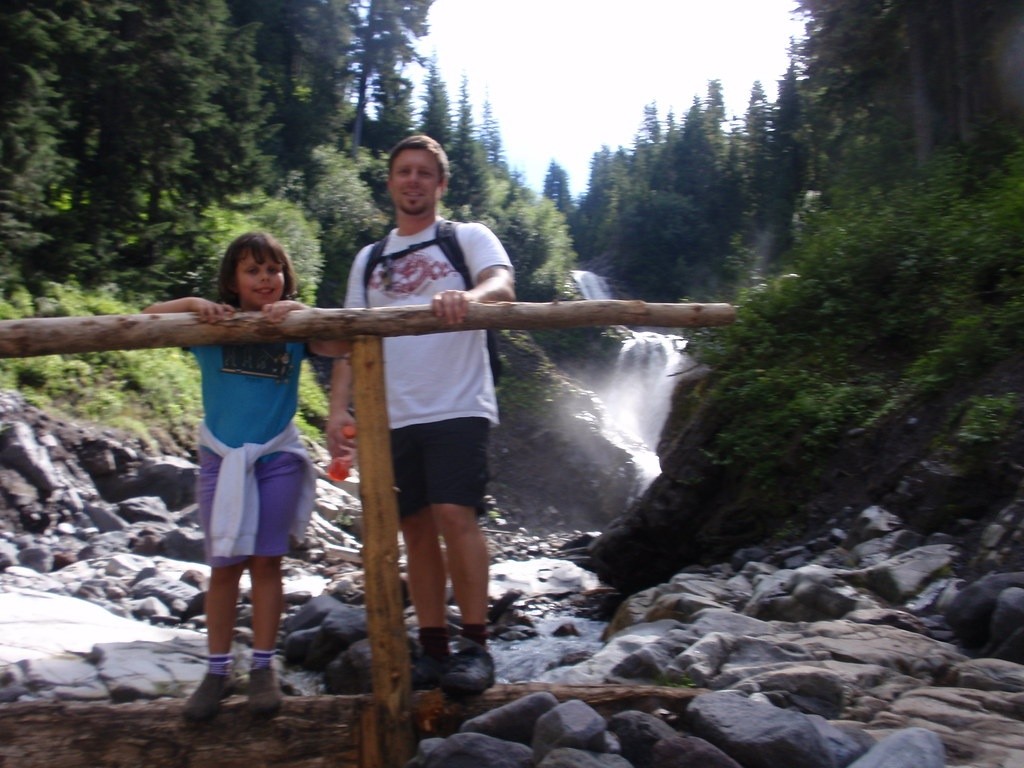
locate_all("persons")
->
[141,233,351,723]
[324,133,514,698]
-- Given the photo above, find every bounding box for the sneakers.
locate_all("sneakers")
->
[440,646,495,690]
[248,666,280,713]
[183,672,233,721]
[412,655,448,689]
